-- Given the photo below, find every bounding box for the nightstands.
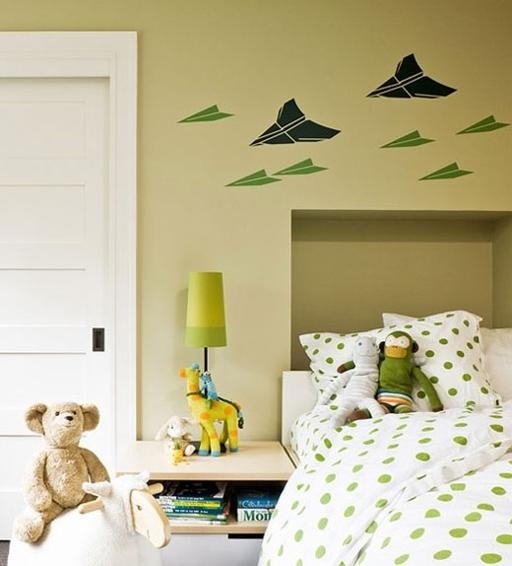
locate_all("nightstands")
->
[132,442,294,538]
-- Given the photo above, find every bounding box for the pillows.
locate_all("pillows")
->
[297,307,512,412]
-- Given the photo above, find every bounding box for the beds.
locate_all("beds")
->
[264,371,512,564]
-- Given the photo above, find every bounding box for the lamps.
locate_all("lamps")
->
[187,269,226,454]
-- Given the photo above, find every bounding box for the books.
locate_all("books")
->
[156,483,280,526]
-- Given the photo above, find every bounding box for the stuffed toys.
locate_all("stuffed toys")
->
[152,414,196,463]
[320,334,385,426]
[12,401,110,542]
[176,361,245,456]
[199,370,219,410]
[338,330,445,421]
